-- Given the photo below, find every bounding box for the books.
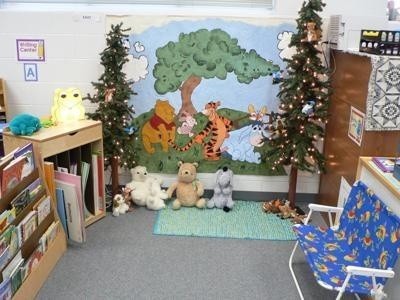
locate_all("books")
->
[54,148,105,245]
[0,140,62,300]
[370,155,400,173]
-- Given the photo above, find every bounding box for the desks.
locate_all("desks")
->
[2,120,107,228]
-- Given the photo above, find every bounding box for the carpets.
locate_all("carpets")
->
[153,199,300,241]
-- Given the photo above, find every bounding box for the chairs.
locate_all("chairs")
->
[288,181,399,300]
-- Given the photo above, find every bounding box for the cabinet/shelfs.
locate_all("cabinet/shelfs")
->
[0,155,68,300]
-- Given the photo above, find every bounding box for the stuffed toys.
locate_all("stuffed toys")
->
[207,165,235,213]
[260,197,313,225]
[126,165,169,211]
[112,194,130,217]
[6,114,41,137]
[41,117,55,130]
[167,160,207,210]
[50,86,86,126]
